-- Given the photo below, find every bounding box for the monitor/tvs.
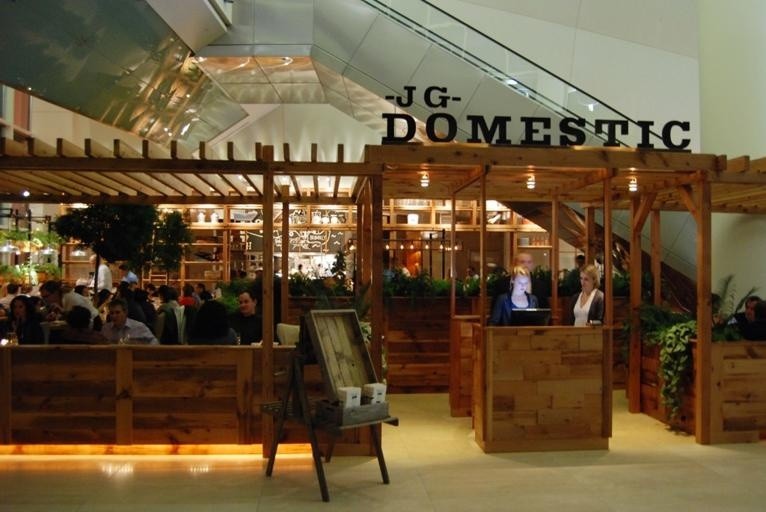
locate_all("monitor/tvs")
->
[510,307,552,326]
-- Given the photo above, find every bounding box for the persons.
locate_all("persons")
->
[86,253,113,292]
[117,264,138,282]
[490,264,539,322]
[575,255,585,266]
[568,265,604,326]
[465,266,479,280]
[491,253,553,322]
[712,293,766,338]
[0,280,262,347]
[254,256,282,324]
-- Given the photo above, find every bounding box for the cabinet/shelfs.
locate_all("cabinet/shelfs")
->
[60,240,230,298]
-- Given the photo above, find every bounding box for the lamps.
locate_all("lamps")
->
[628,179,638,193]
[420,174,430,188]
[527,177,535,189]
